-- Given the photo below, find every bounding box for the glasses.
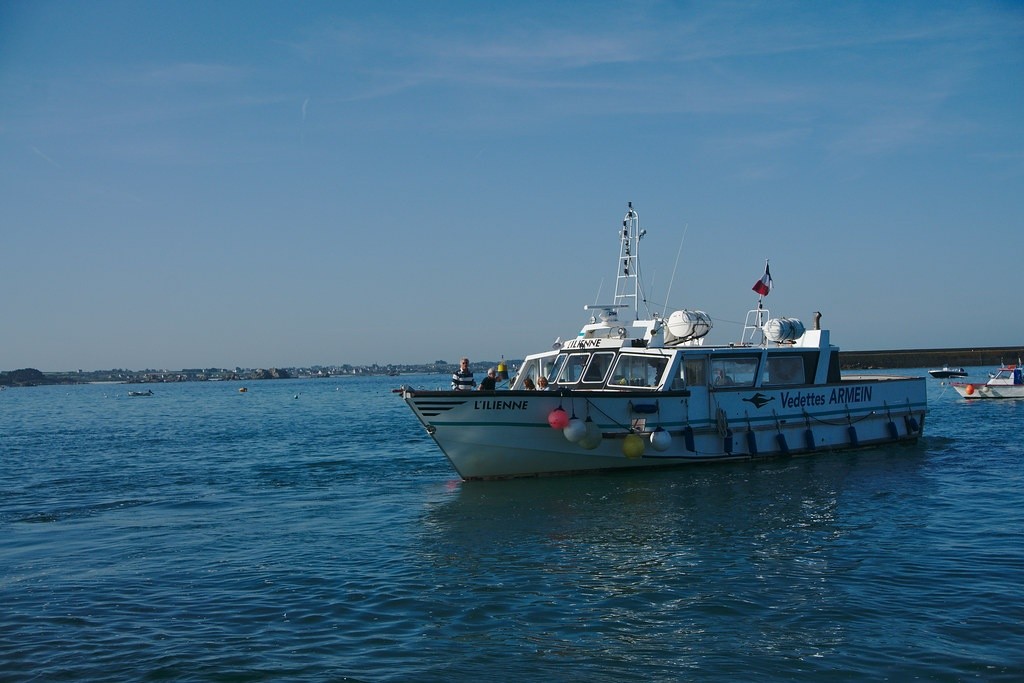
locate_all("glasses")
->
[462,362,468,365]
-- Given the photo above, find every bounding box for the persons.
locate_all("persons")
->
[523,376,552,392]
[451,358,477,392]
[477,367,497,390]
[714,368,735,386]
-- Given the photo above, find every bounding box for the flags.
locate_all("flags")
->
[751,263,773,295]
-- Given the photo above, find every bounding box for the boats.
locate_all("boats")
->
[128,391,152,397]
[393,201,925,477]
[928,364,968,378]
[940,357,1024,399]
